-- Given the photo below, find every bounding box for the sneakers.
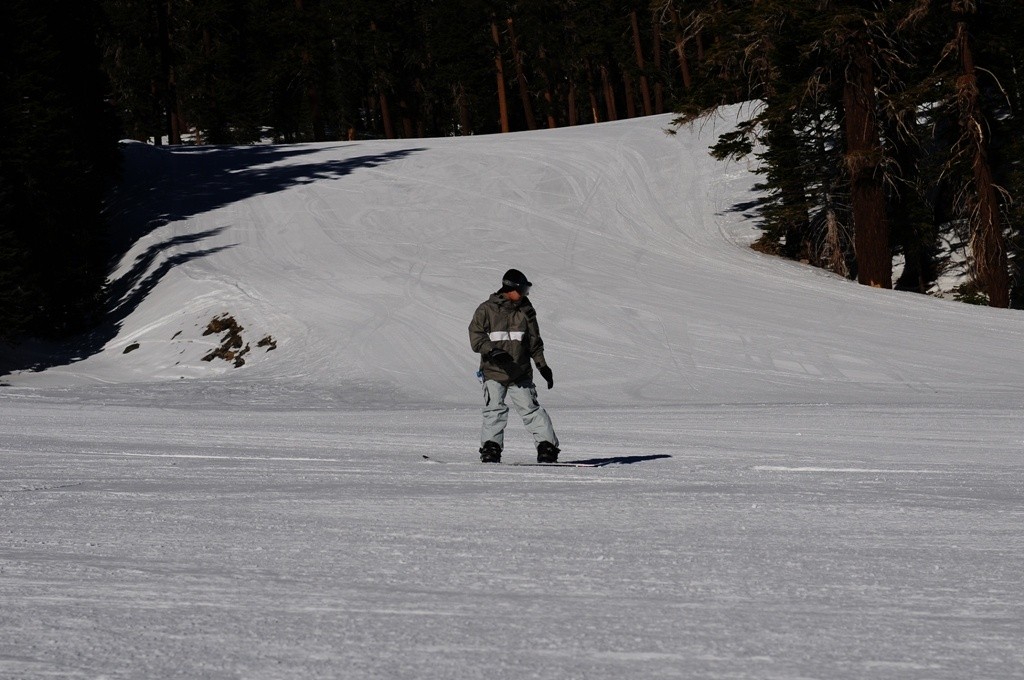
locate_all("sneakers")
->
[537,447,556,463]
[480,445,500,462]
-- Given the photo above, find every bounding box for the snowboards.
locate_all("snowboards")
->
[422,454,612,468]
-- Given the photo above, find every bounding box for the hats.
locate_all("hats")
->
[502,269,532,292]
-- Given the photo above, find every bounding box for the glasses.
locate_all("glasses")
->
[515,283,530,296]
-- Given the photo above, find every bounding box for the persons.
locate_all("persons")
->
[468,269,560,463]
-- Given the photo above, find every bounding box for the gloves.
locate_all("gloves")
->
[487,349,514,369]
[539,366,554,389]
